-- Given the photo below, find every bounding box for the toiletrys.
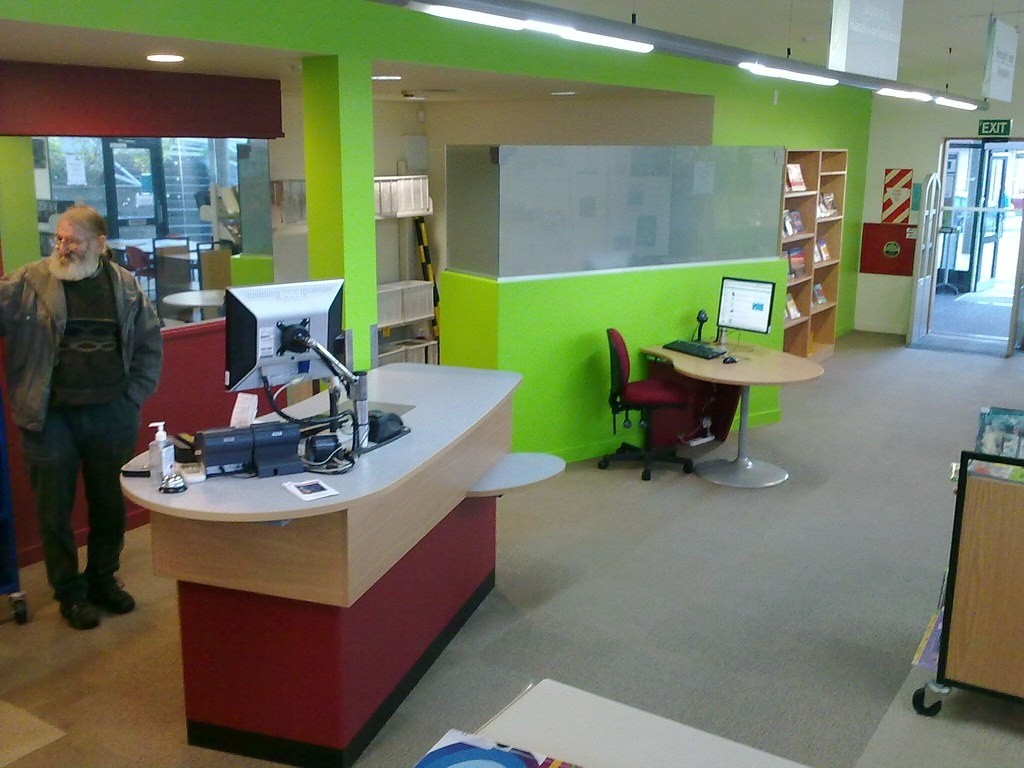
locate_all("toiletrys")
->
[148,420,174,485]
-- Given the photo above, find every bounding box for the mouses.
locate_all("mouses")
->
[723,357,737,363]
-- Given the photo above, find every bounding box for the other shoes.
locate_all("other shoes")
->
[8,592,27,625]
[86,578,135,614]
[59,590,99,629]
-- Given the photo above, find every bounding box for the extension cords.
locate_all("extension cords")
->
[689,434,715,447]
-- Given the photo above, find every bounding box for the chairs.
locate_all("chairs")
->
[598,329,694,482]
[124,245,156,297]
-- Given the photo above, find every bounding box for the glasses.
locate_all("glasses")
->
[55,236,89,251]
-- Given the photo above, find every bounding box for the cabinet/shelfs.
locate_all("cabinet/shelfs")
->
[152,237,232,324]
[912,407,1024,715]
[778,148,850,363]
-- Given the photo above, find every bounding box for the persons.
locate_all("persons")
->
[0,204,164,631]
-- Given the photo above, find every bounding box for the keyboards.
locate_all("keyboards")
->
[663,339,727,360]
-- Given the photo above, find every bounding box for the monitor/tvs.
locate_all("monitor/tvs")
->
[717,278,777,335]
[225,279,345,390]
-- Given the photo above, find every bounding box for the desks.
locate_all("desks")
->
[475,679,809,768]
[120,363,568,768]
[106,238,210,290]
[641,339,825,489]
